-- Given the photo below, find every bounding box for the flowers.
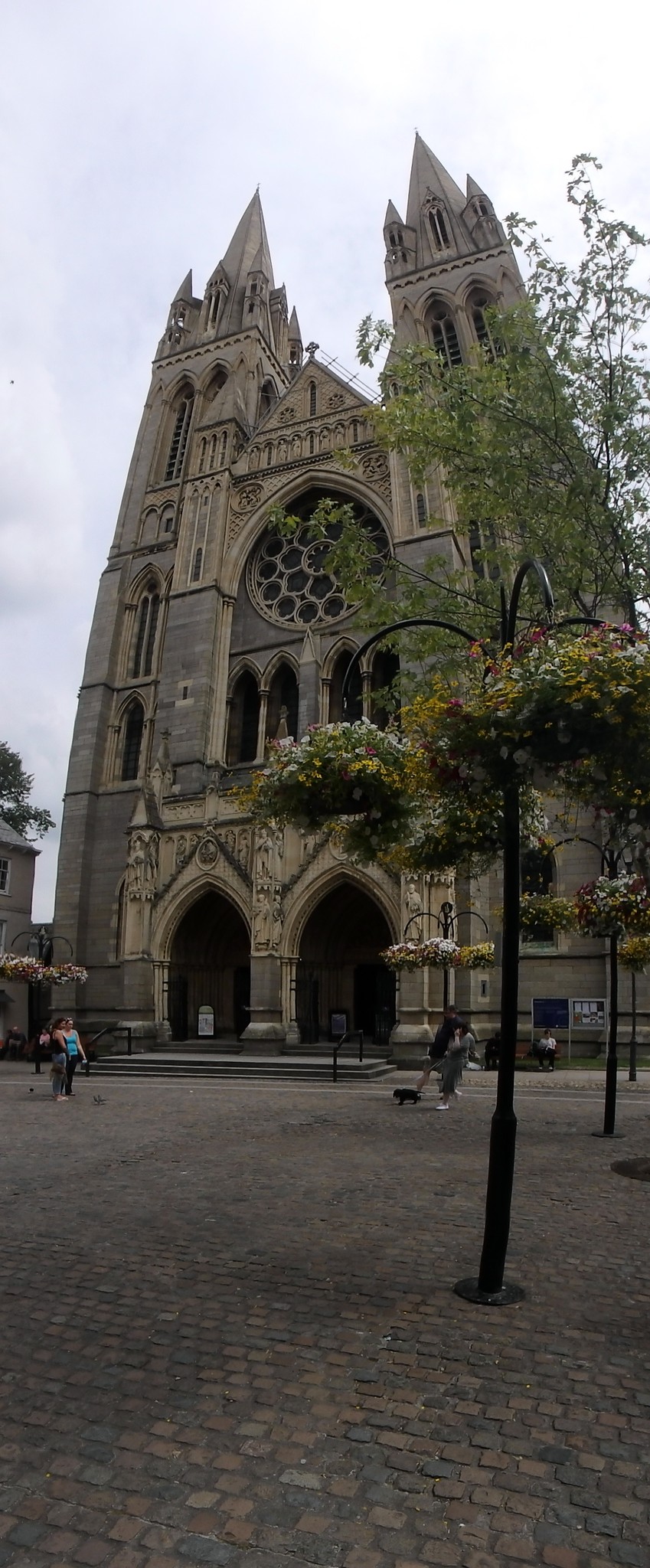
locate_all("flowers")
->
[226,618,650,975]
[0,951,89,988]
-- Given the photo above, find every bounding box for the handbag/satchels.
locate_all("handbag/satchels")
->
[533,1041,539,1056]
[51,1054,66,1073]
[527,1050,533,1056]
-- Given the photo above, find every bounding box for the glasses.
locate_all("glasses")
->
[444,1011,451,1015]
[65,1017,72,1021]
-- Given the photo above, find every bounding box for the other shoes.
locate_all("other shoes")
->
[454,1090,462,1103]
[539,1066,543,1069]
[485,1064,490,1071]
[548,1065,553,1069]
[436,1103,448,1109]
[57,1097,69,1101]
[67,1093,75,1096]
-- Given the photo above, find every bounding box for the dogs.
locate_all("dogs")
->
[392,1088,426,1107]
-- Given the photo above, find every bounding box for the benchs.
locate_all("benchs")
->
[490,1041,562,1069]
[0,1039,5,1048]
[28,1037,97,1062]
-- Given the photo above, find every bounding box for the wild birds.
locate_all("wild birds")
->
[29,1088,34,1093]
[93,1094,107,1106]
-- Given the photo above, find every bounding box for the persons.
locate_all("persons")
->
[253,894,270,948]
[537,1029,557,1070]
[417,1004,462,1100]
[60,1017,87,1096]
[40,1027,50,1047]
[51,1017,69,1102]
[6,1026,27,1061]
[125,840,158,891]
[0,1030,14,1060]
[484,1031,501,1071]
[272,895,283,947]
[434,1020,470,1110]
[404,884,423,945]
